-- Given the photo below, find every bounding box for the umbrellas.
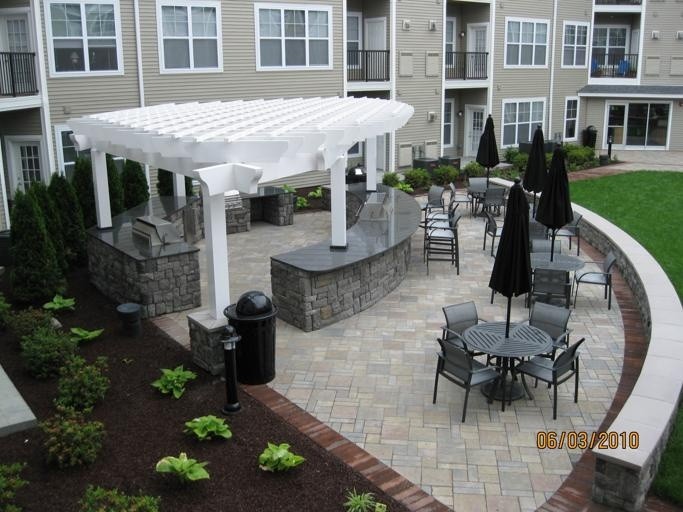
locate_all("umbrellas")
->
[521,122,549,220]
[475,114,499,218]
[536,140,574,307]
[481,176,532,402]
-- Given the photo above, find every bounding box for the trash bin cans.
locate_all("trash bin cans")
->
[346,167,367,202]
[224,290,278,385]
[582,125,598,150]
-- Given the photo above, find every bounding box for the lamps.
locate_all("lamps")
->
[457,110,463,118]
[459,30,466,38]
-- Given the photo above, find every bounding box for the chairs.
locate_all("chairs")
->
[419,181,617,310]
[433,300,585,422]
